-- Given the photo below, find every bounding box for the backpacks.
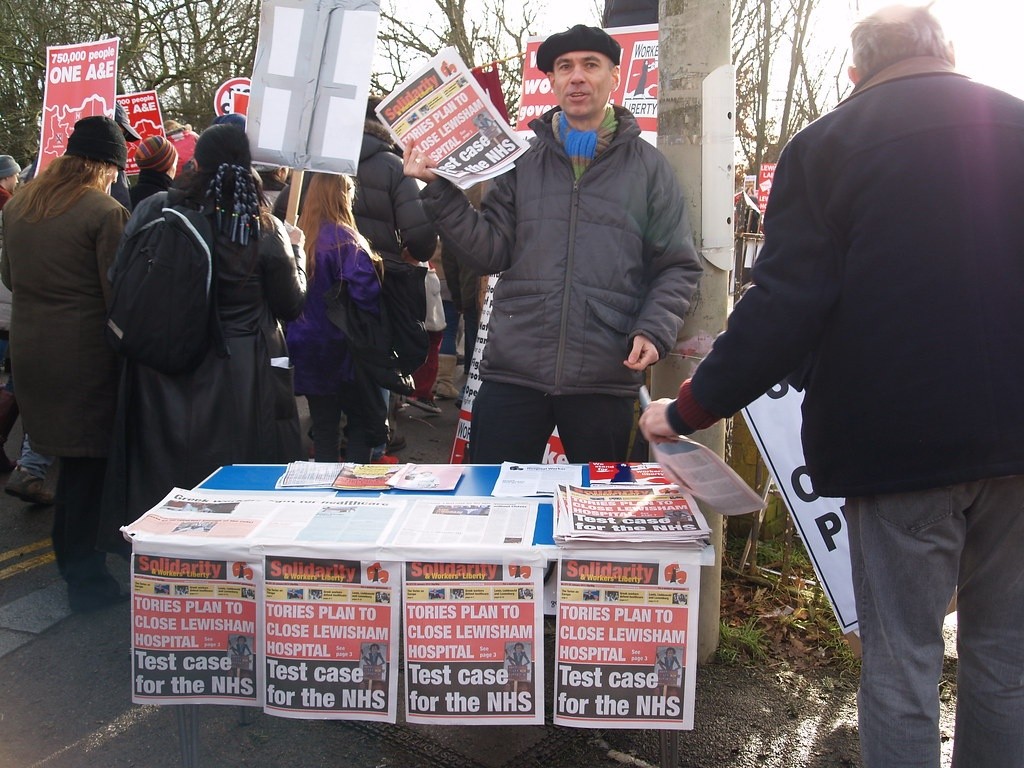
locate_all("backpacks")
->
[102,188,229,378]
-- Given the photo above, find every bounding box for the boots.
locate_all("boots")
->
[0,390,20,473]
[431,353,459,398]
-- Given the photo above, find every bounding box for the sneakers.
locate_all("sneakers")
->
[5,465,53,506]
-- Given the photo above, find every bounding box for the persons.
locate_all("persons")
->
[0,97,499,471]
[640,4,1024,768]
[287,173,386,468]
[402,24,705,465]
[103,125,307,595]
[0,116,132,612]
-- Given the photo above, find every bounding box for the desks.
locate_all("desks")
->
[119,463,710,768]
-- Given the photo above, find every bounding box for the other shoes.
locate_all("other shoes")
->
[455,352,465,365]
[73,581,131,613]
[309,440,345,463]
[370,455,399,464]
[386,431,406,451]
[407,395,443,413]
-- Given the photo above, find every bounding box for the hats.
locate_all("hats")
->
[536,24,620,74]
[66,117,126,171]
[0,155,22,178]
[114,100,142,142]
[135,135,176,174]
